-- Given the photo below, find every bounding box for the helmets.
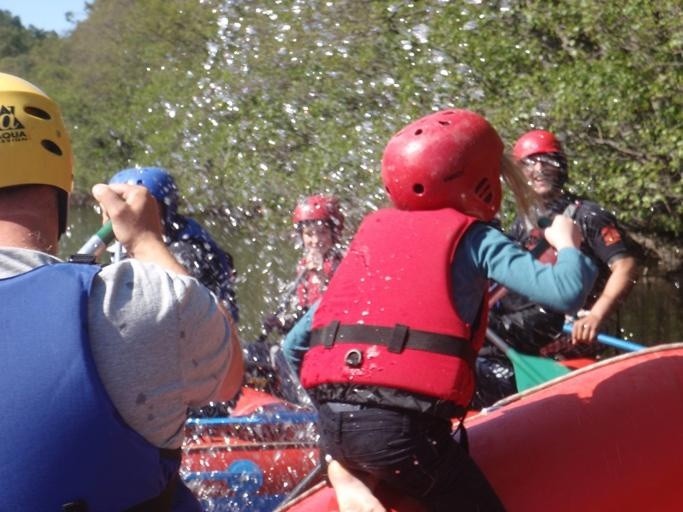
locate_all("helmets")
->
[382,108,505,221]
[0,72,73,235]
[514,129,562,161]
[108,167,179,229]
[291,195,344,232]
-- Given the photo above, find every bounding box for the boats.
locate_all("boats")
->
[181,340,683,511]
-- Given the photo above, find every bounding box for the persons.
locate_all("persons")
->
[469,129,642,411]
[108,164,240,418]
[244,191,348,409]
[284,108,601,511]
[2,73,246,511]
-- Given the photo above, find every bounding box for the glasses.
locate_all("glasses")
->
[522,153,562,168]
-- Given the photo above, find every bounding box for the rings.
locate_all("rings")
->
[583,325,591,329]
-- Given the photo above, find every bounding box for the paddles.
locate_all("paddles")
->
[486,328,573,395]
